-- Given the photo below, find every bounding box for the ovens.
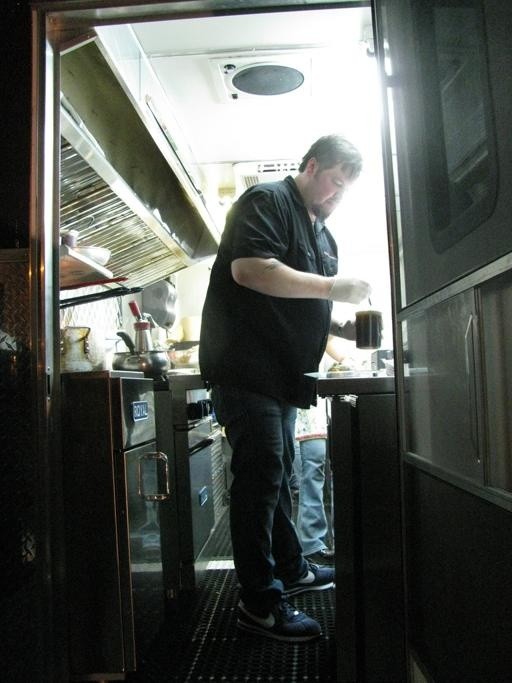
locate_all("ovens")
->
[168,389,214,592]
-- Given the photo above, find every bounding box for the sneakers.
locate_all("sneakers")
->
[282,563,336,600]
[235,599,325,644]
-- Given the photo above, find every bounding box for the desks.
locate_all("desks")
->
[318,365,414,681]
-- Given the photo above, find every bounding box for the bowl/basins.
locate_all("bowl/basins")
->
[62,244,111,265]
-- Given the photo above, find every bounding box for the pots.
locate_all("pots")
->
[110,332,170,376]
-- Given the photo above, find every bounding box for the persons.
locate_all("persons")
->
[294,336,351,562]
[199,136,383,643]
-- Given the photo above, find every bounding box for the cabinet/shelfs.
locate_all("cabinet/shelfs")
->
[58,369,173,679]
[160,371,215,613]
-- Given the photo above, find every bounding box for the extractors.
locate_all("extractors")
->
[51,27,225,289]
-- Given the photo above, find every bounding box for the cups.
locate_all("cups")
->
[356,309,382,352]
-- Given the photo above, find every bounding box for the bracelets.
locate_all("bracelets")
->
[327,275,338,299]
